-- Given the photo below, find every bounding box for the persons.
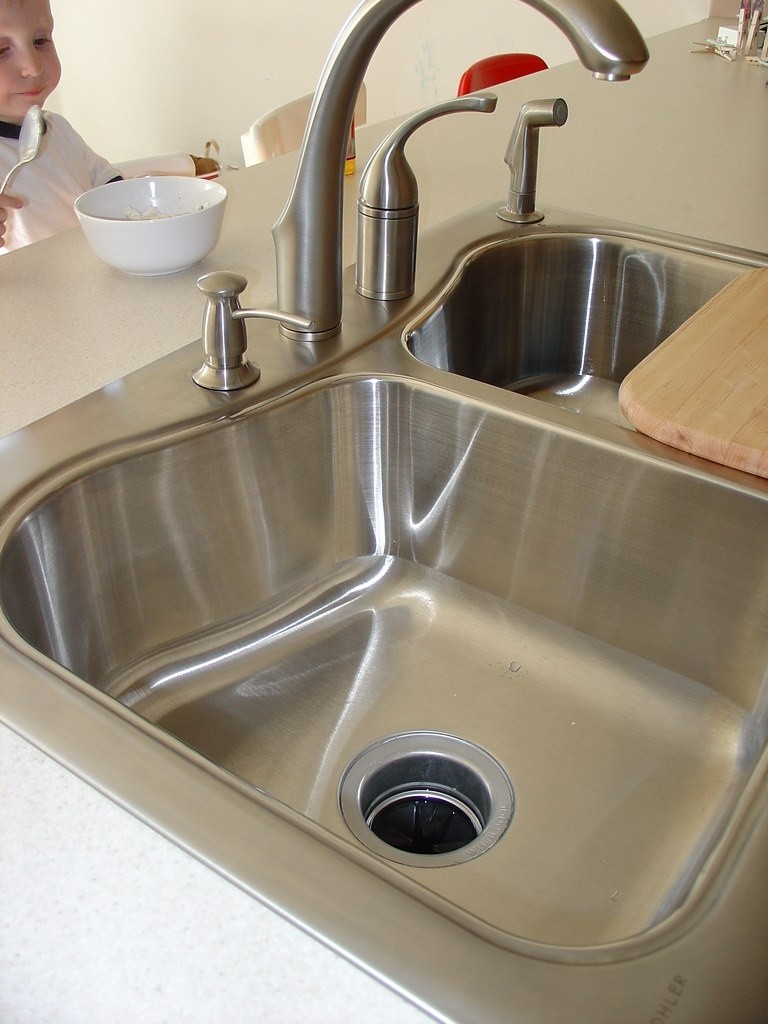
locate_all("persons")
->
[0,0,125,248]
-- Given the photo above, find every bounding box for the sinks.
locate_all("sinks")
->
[0,362,768,1021]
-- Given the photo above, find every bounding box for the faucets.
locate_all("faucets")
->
[272,2,650,347]
[230,199,767,495]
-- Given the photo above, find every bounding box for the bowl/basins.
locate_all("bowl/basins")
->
[71,174,229,278]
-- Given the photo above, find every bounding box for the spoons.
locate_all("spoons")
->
[0,103,43,196]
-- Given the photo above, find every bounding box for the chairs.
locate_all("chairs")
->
[456,53,550,96]
[109,153,222,181]
[240,80,366,167]
